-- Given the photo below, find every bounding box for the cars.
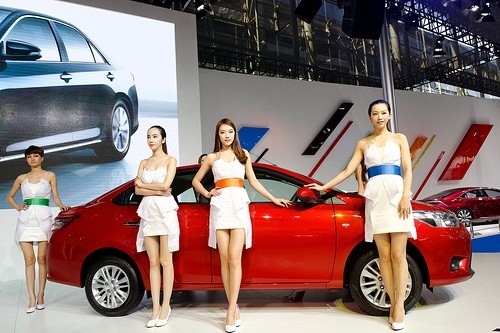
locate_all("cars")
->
[416,185,500,228]
[45,162,475,316]
[0,5,140,162]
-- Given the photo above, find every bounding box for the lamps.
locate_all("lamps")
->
[432,35,446,59]
[465,0,496,23]
[194,0,205,12]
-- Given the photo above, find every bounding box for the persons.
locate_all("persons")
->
[7,146,71,313]
[132,126,180,328]
[191,119,293,333]
[304,99,417,332]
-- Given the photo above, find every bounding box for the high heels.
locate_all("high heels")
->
[225,312,236,332]
[236,308,241,327]
[392,308,404,330]
[26,295,36,312]
[37,300,44,310]
[146,306,161,327]
[388,309,393,324]
[155,305,171,325]
